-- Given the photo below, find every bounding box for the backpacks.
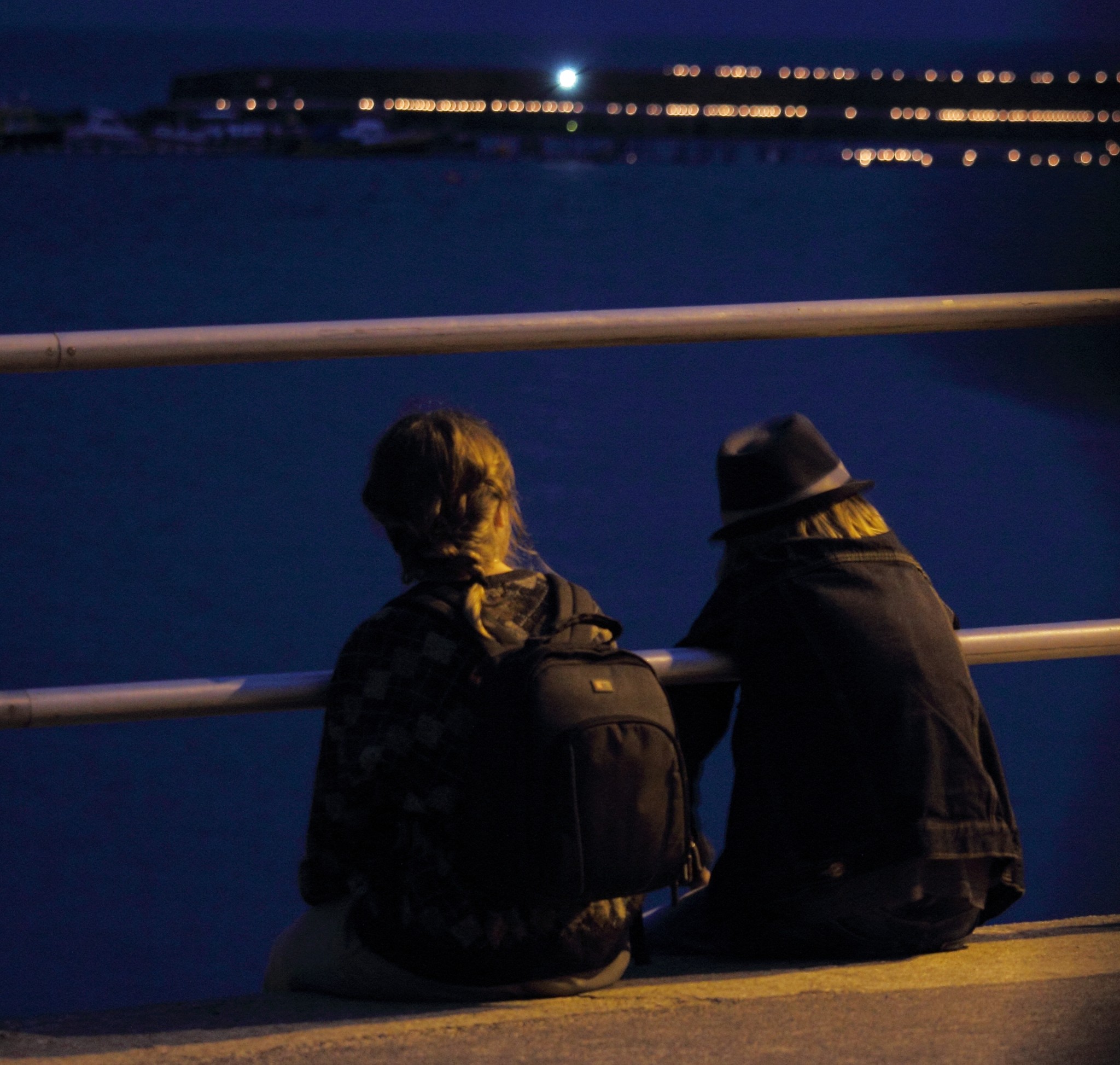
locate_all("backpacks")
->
[384,572,695,906]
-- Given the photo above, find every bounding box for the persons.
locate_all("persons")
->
[264,408,706,1009]
[667,415,1028,971]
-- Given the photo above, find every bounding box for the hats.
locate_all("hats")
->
[706,410,877,543]
